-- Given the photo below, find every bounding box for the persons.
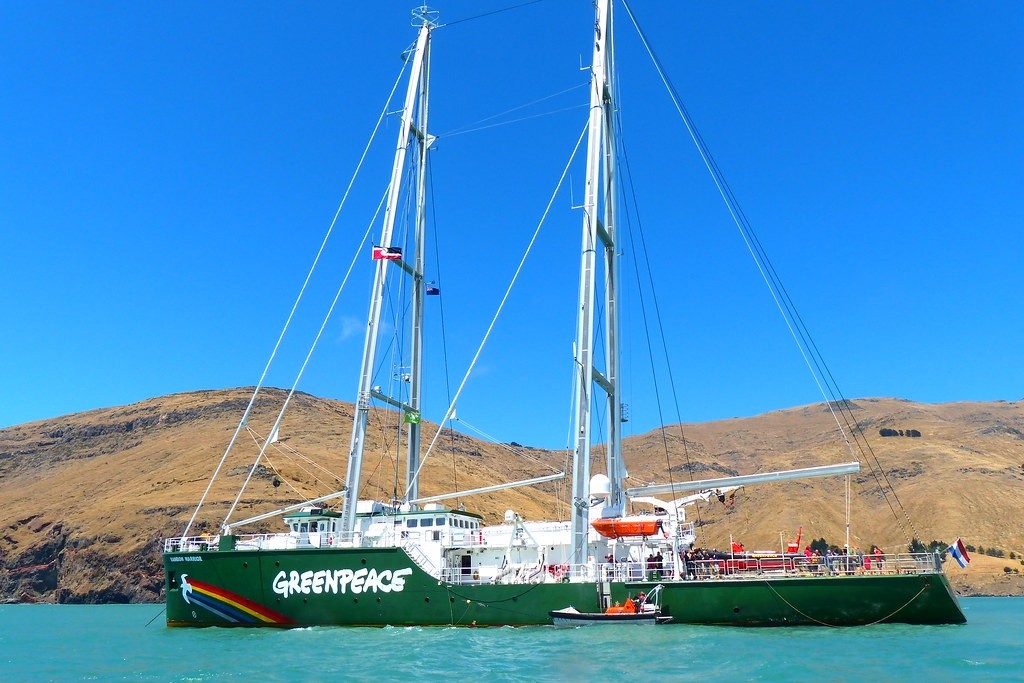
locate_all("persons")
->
[607,547,726,582]
[804,546,883,577]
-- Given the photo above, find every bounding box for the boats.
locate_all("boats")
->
[547,584,675,626]
[591,517,663,539]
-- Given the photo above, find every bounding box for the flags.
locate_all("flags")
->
[949,538,970,568]
[732,540,744,552]
[372,245,402,260]
[796,527,803,543]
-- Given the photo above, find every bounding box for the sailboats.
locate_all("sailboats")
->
[160,0,970,633]
